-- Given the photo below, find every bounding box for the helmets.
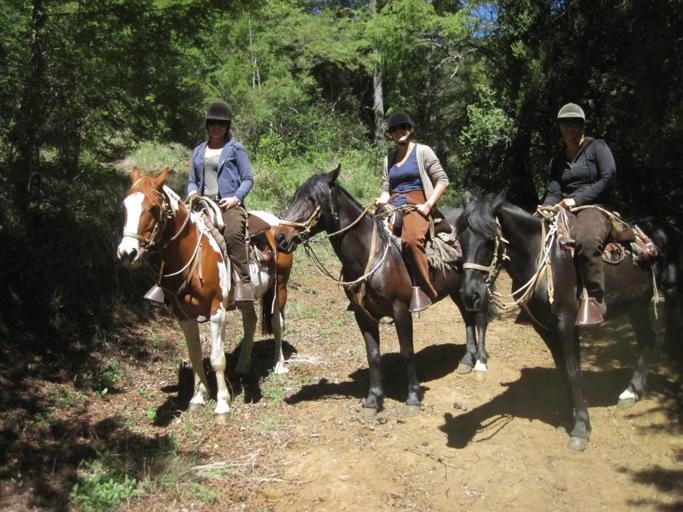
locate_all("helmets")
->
[385,111,414,134]
[556,102,585,123]
[206,100,233,122]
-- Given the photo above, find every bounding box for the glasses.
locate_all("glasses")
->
[208,120,225,126]
[390,123,410,131]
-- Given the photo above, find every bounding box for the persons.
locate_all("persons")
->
[186,101,253,294]
[540,102,618,316]
[373,111,449,298]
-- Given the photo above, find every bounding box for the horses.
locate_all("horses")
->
[272,161,500,423]
[113,162,296,427]
[451,182,682,453]
